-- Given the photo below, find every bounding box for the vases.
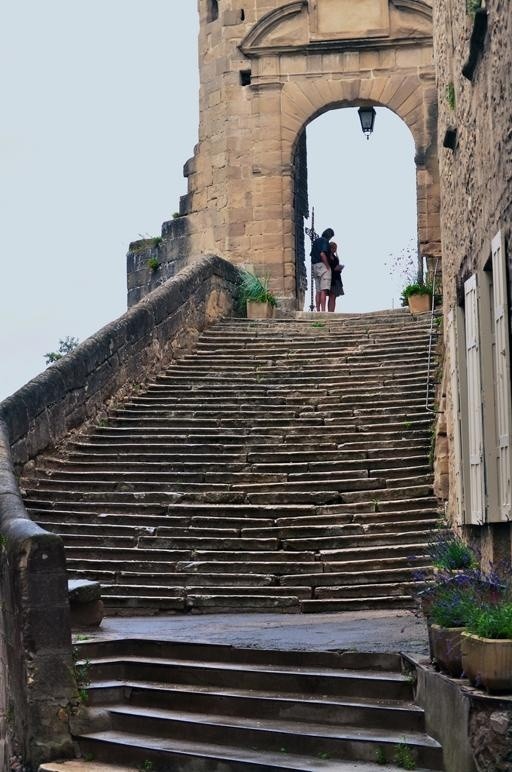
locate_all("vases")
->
[430,623,512,697]
[407,293,431,313]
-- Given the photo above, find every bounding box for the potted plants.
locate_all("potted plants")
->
[236,267,278,319]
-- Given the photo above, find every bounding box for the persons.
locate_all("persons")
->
[326,241,344,313]
[309,228,335,313]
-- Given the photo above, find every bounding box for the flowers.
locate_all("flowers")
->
[384,238,443,306]
[394,506,512,689]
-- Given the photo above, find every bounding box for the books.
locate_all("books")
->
[334,264,344,272]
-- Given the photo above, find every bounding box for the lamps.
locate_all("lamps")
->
[358,106,376,140]
